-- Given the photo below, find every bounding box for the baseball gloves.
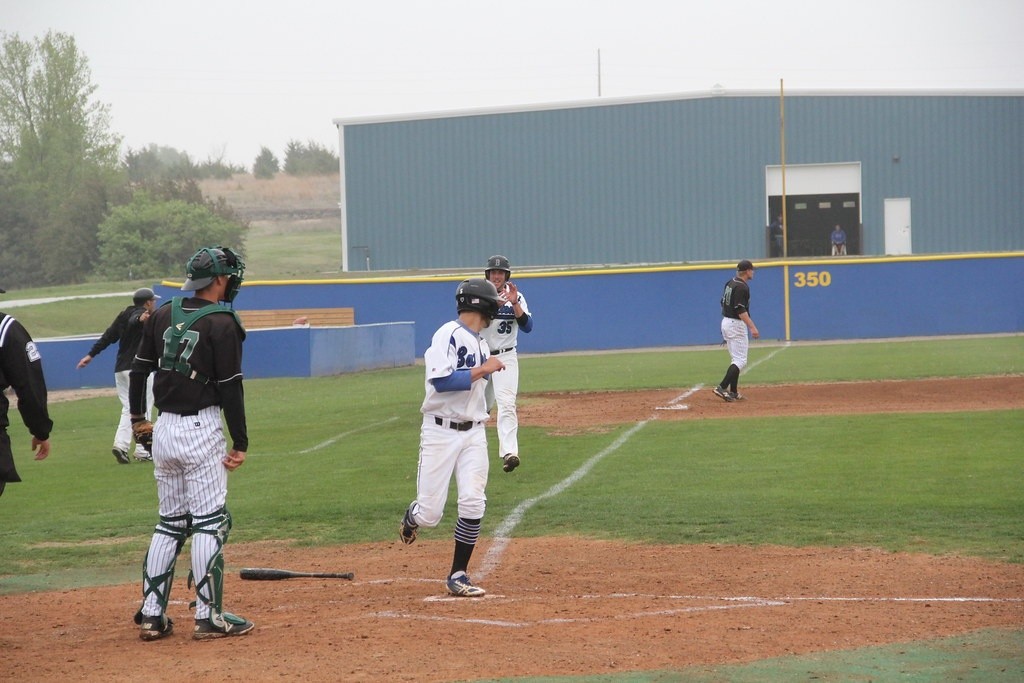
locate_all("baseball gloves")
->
[131,421,156,457]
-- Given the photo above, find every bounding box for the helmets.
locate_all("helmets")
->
[455,278,500,320]
[133,287,162,307]
[484,255,511,281]
[181,249,228,291]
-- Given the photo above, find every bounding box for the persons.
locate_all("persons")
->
[831,225,847,256]
[128,248,254,641]
[0,289,51,497]
[474,256,533,473]
[770,214,784,257]
[712,260,759,402]
[399,277,506,597]
[76,288,160,463]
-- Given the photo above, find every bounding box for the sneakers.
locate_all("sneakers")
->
[503,454,519,473]
[713,385,732,402]
[112,446,130,464]
[446,574,486,597]
[399,501,419,545]
[141,615,174,640]
[192,617,254,640]
[729,392,747,400]
[133,455,153,461]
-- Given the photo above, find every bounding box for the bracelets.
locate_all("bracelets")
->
[512,302,517,305]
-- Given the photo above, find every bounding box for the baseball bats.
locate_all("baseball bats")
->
[239,567,355,583]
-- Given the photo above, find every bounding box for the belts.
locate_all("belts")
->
[435,416,480,431]
[490,346,516,355]
[158,410,198,418]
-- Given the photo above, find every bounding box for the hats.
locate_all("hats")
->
[737,260,754,271]
